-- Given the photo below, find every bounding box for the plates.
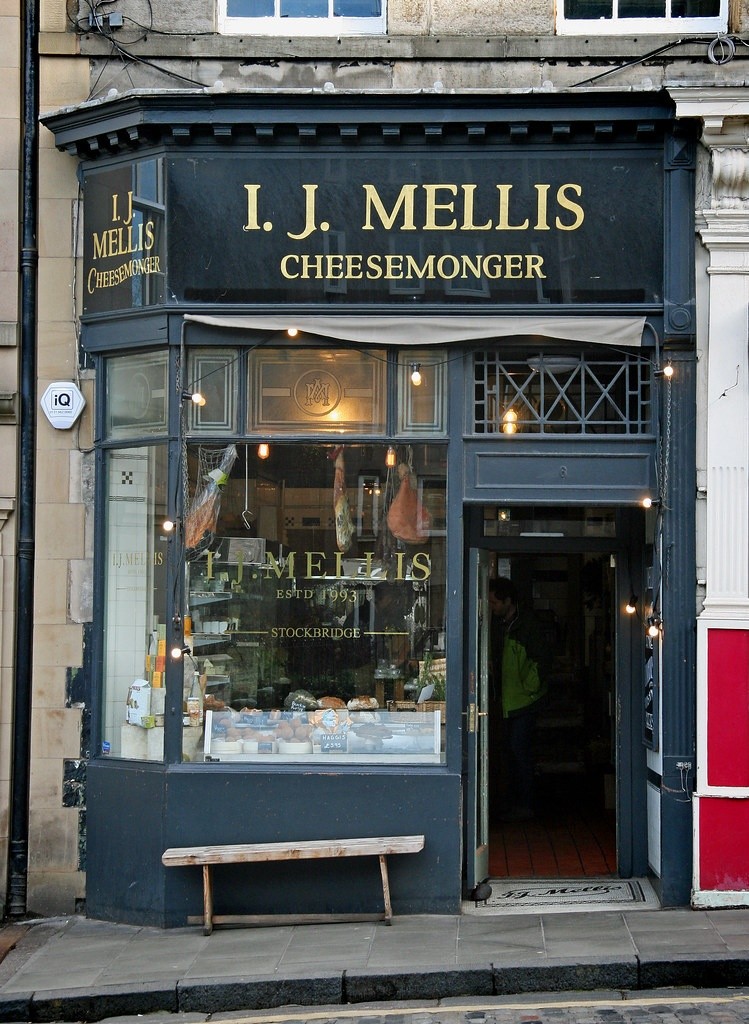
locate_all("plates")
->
[210,741,242,754]
[279,742,313,753]
[244,741,277,754]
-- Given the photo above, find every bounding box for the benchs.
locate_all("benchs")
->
[162,836,426,936]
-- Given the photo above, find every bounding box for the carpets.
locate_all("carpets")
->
[468,879,662,916]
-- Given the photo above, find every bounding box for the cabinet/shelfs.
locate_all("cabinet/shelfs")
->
[184,559,262,689]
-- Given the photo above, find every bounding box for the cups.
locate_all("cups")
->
[193,611,228,635]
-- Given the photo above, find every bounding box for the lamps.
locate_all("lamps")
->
[527,354,580,375]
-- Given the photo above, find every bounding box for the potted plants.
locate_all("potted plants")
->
[416,653,446,724]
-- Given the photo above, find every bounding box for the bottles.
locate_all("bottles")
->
[184,615,192,635]
[190,672,203,725]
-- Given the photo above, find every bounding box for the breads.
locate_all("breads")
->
[204,690,380,733]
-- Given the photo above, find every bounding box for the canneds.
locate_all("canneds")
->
[184,615,191,636]
[183,712,190,727]
[156,713,164,727]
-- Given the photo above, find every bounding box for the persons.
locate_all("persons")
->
[342,583,394,669]
[488,577,553,823]
